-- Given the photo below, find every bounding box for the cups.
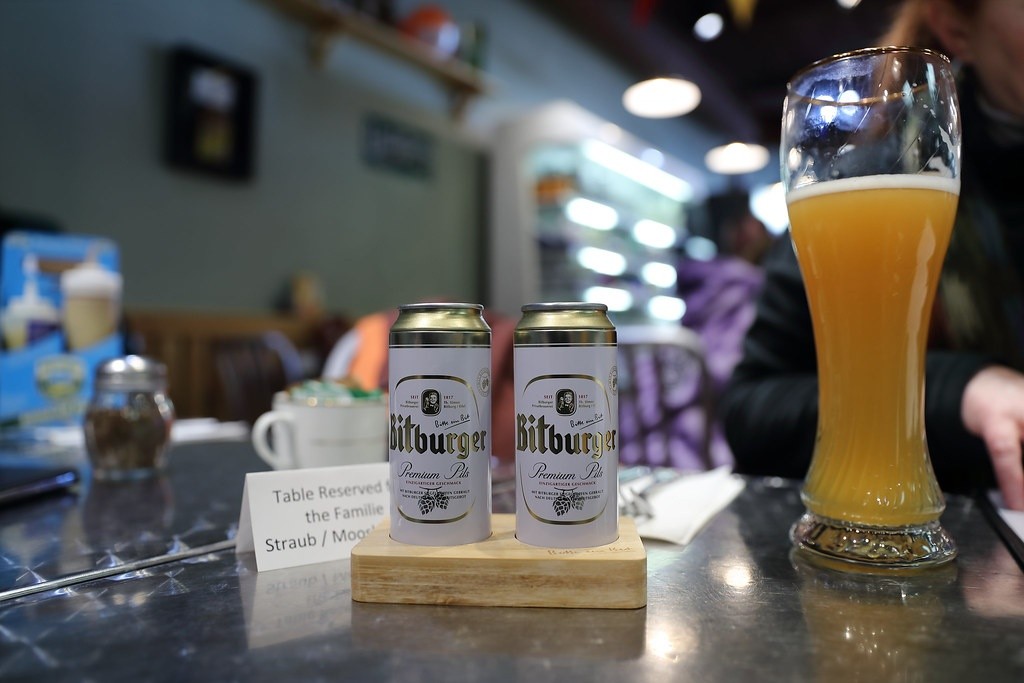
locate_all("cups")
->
[253,389,390,469]
[61,269,123,353]
[780,46,962,577]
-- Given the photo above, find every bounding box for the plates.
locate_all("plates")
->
[0,465,81,512]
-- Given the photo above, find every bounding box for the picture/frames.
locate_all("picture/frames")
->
[163,43,260,185]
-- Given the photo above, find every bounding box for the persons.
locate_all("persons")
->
[722,0,1024,516]
[671,184,777,397]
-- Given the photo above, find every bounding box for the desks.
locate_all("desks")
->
[0,420,1024,683]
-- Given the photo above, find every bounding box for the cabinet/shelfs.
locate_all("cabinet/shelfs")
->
[483,101,710,353]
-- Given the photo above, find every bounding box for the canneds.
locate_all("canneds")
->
[512,301,618,548]
[388,302,494,546]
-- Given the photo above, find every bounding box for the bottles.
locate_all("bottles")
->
[85,353,175,485]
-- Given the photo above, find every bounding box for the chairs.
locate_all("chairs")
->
[321,300,518,461]
[122,301,330,413]
[608,321,723,467]
